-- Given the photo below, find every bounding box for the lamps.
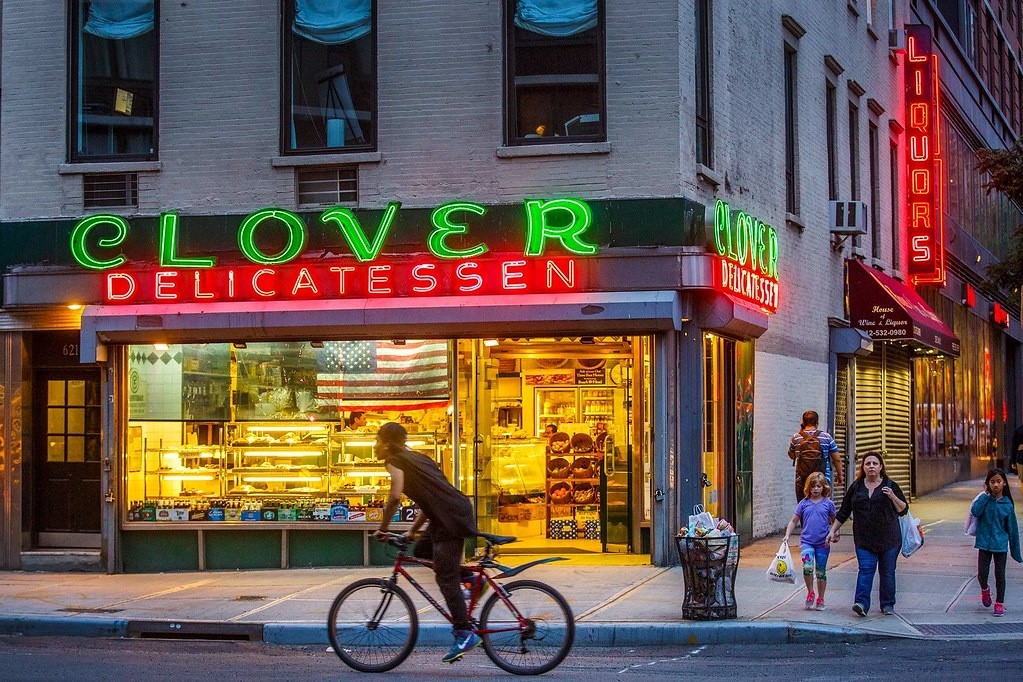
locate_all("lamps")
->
[154,344,170,350]
[580,336,596,344]
[310,341,324,348]
[483,339,500,347]
[393,338,406,345]
[233,342,247,349]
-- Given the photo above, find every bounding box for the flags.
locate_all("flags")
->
[317,340,448,401]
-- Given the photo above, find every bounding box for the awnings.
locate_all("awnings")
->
[849,258,960,358]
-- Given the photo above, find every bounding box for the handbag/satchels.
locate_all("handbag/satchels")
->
[765,540,796,584]
[898,510,924,558]
[963,490,987,536]
[688,504,715,535]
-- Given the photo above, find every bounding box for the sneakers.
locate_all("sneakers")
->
[470,577,490,606]
[852,602,867,616]
[882,606,894,615]
[993,603,1005,616]
[981,584,992,607]
[444,629,482,661]
[816,597,826,611]
[805,590,815,606]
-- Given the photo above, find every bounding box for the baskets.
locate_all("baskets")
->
[546,431,614,504]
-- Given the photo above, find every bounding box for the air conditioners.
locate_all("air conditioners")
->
[888,28,909,55]
[828,200,869,235]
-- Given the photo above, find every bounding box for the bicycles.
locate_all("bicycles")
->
[327,530,576,675]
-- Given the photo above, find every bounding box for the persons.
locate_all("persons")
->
[1012,425,1023,483]
[826,452,909,616]
[918,418,999,456]
[971,470,1023,616]
[339,411,366,462]
[544,425,558,438]
[782,472,840,610]
[374,423,490,663]
[788,411,843,528]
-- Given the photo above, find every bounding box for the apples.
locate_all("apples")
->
[551,488,569,499]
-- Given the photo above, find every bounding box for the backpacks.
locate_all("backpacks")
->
[797,430,826,477]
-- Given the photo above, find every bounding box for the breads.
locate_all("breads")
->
[233,431,302,443]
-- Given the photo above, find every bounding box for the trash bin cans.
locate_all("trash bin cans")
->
[676,533,740,621]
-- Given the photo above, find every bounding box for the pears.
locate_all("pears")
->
[551,440,569,451]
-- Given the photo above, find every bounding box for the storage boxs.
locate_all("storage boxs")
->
[498,506,526,522]
[517,503,545,520]
[128,500,423,522]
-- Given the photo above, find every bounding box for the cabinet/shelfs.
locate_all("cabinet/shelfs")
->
[143,428,233,499]
[545,446,606,540]
[223,422,336,498]
[326,429,439,498]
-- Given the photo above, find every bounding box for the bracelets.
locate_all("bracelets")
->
[379,528,388,533]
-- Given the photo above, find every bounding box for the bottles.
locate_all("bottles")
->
[582,391,613,439]
[543,400,575,413]
[539,418,574,431]
[128,499,414,522]
